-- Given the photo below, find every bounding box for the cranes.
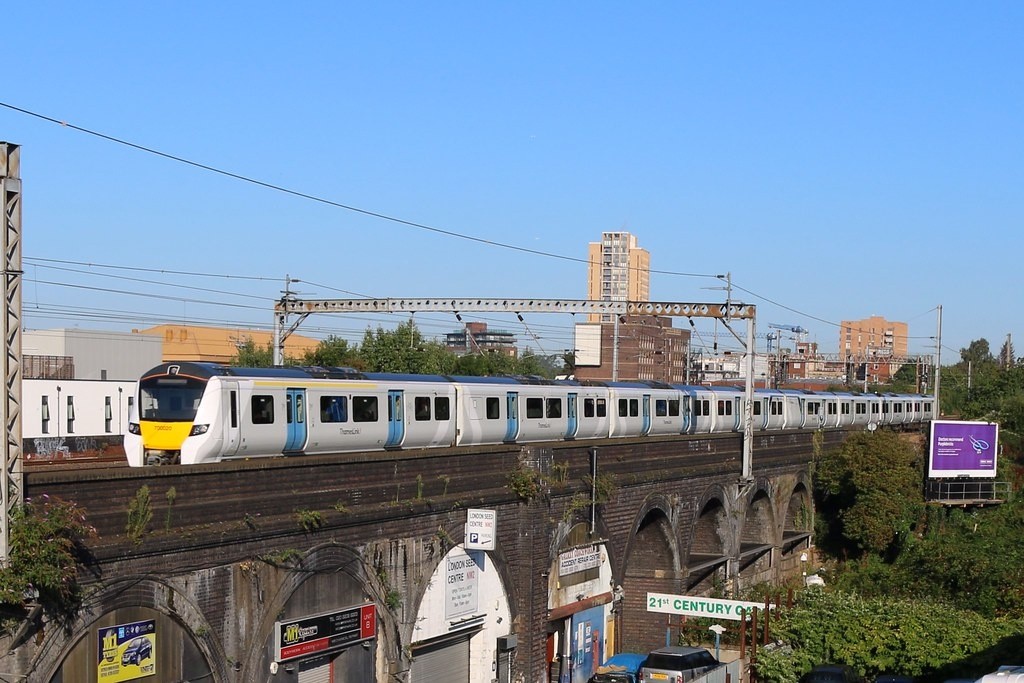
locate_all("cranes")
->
[692,331,781,353]
[768,323,807,343]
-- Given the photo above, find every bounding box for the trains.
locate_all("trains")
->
[123,360,940,466]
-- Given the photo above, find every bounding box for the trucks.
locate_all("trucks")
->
[593,653,647,683]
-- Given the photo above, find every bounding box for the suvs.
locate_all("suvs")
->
[800,664,866,683]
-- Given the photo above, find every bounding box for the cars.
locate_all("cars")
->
[876,675,912,683]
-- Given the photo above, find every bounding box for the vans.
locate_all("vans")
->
[639,647,719,683]
[973,665,1024,683]
